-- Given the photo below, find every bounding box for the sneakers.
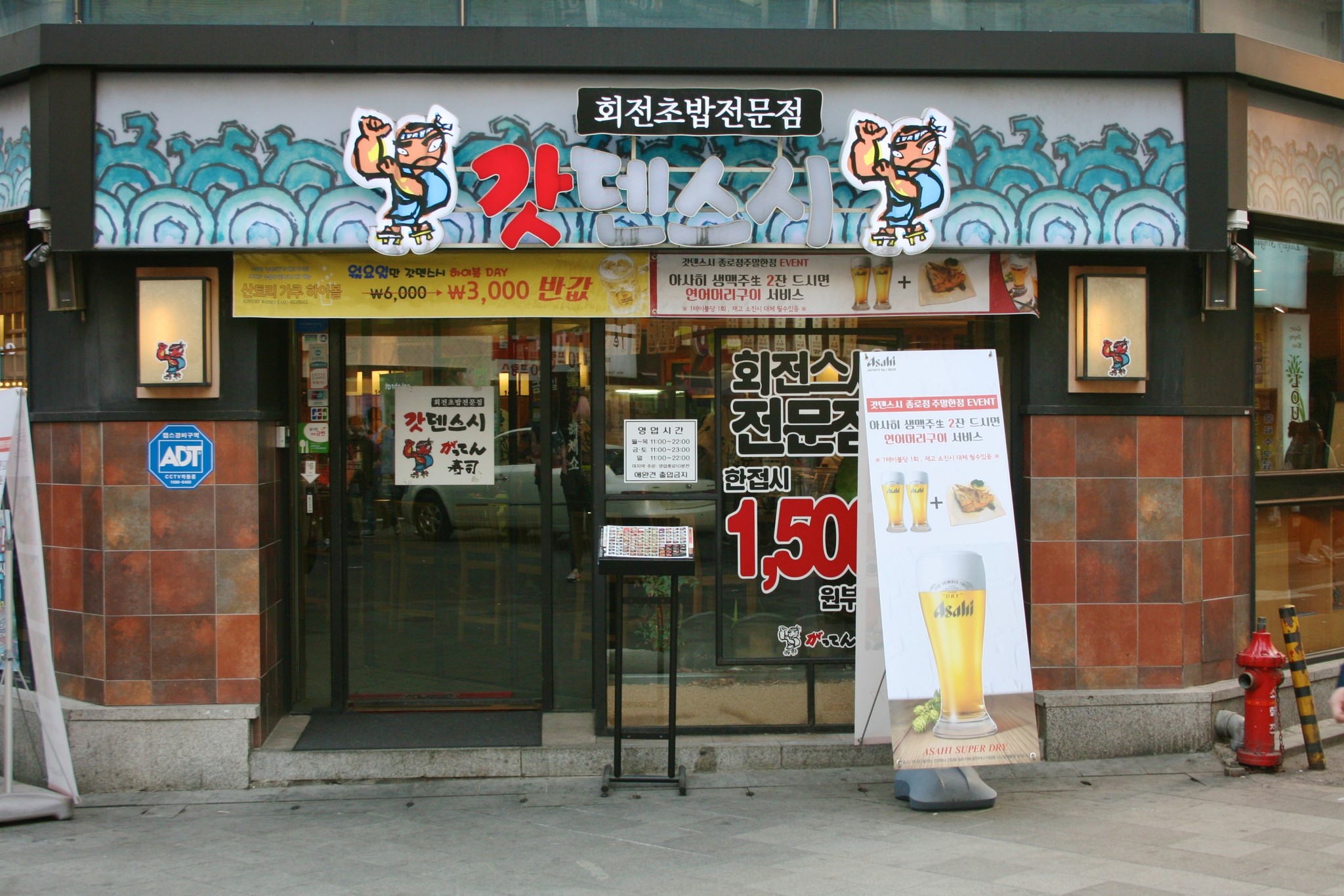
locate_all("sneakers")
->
[567,569,581,582]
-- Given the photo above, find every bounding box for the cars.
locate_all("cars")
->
[400,425,726,552]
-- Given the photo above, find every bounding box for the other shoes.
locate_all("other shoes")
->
[395,521,402,535]
[360,530,378,537]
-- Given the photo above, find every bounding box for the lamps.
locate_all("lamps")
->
[1274,304,1288,313]
[1068,265,1149,394]
[135,266,220,399]
[1228,244,1256,267]
[23,244,51,268]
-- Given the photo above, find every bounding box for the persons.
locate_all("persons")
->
[531,367,592,583]
[346,407,409,538]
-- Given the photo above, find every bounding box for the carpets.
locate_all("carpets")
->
[291,711,543,751]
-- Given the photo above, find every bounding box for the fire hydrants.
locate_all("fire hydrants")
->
[1235,615,1289,772]
